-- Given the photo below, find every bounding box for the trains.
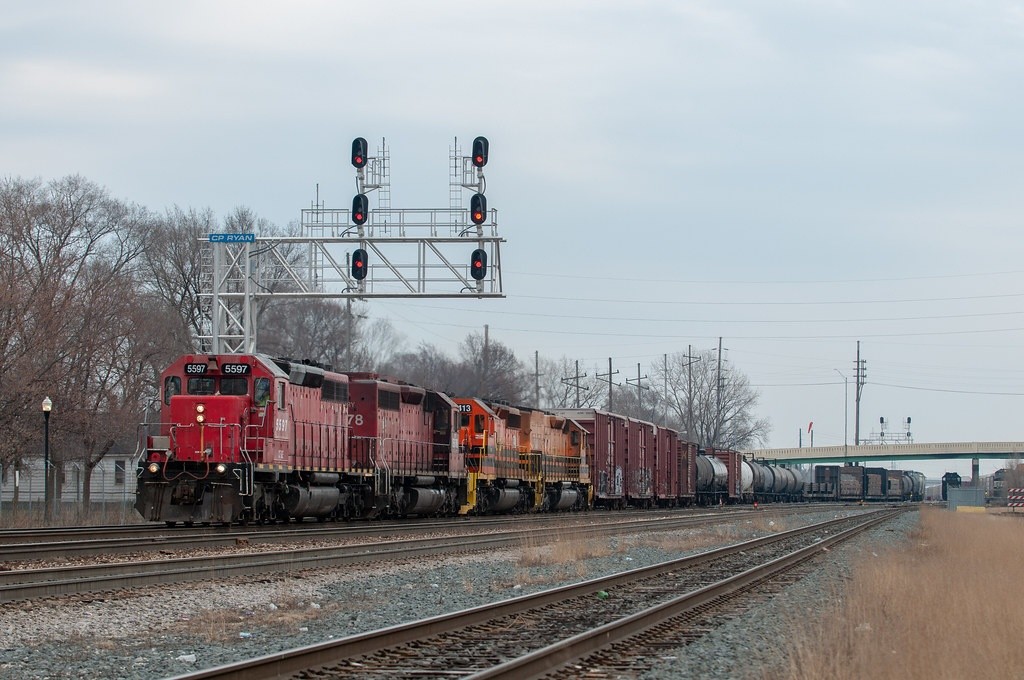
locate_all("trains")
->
[135,353,926,528]
[942,472,961,501]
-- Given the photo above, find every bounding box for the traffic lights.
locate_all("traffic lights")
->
[471,249,487,280]
[352,249,368,279]
[352,194,368,225]
[472,136,489,167]
[352,137,368,168]
[471,194,487,224]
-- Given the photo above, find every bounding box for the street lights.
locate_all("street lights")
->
[42,397,52,523]
[834,369,847,457]
